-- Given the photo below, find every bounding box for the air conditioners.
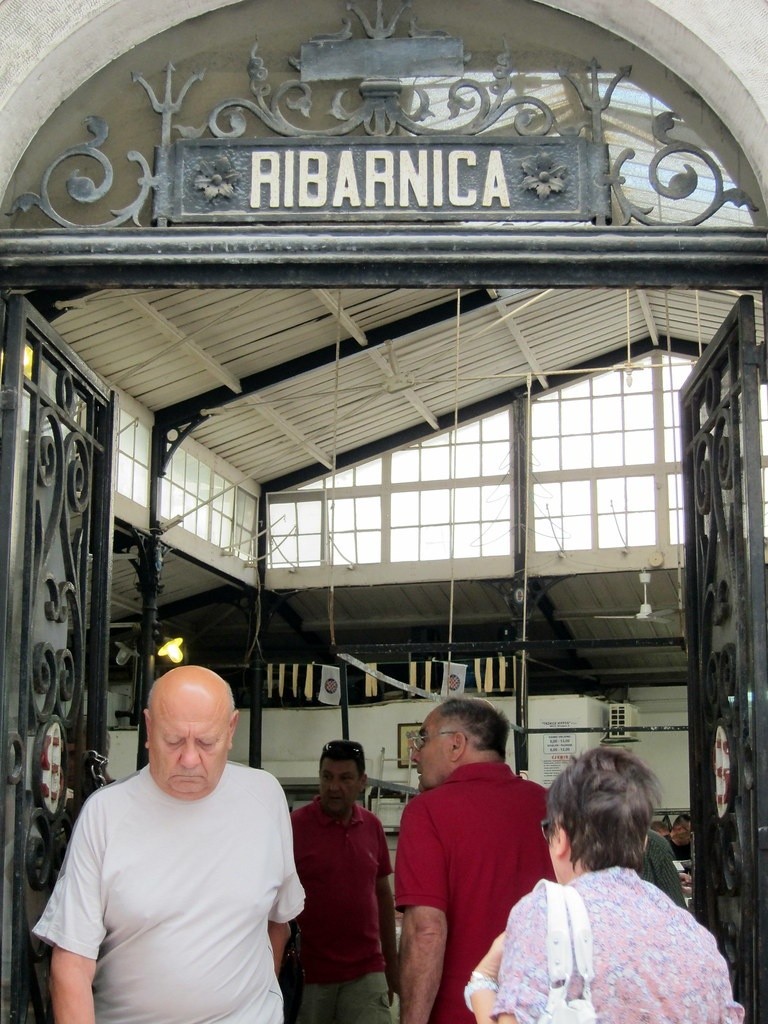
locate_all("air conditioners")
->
[609,703,640,740]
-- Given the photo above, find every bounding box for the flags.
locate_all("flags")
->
[441,662,468,697]
[319,665,342,705]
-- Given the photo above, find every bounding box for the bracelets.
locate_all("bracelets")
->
[465,978,495,1012]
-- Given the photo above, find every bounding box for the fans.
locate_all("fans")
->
[593,571,678,623]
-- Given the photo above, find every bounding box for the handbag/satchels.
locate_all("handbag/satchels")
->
[534,877,598,1024]
[279,920,302,1024]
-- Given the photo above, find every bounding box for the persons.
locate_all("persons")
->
[31,666,306,1024]
[395,702,556,1024]
[665,814,690,860]
[639,830,686,910]
[290,741,397,1024]
[650,815,669,836]
[463,747,745,1024]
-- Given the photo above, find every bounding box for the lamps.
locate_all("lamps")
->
[115,628,142,666]
[156,620,183,663]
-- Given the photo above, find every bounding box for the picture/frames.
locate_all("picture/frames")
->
[397,723,423,767]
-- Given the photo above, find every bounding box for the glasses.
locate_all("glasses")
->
[540,820,557,837]
[411,730,454,748]
